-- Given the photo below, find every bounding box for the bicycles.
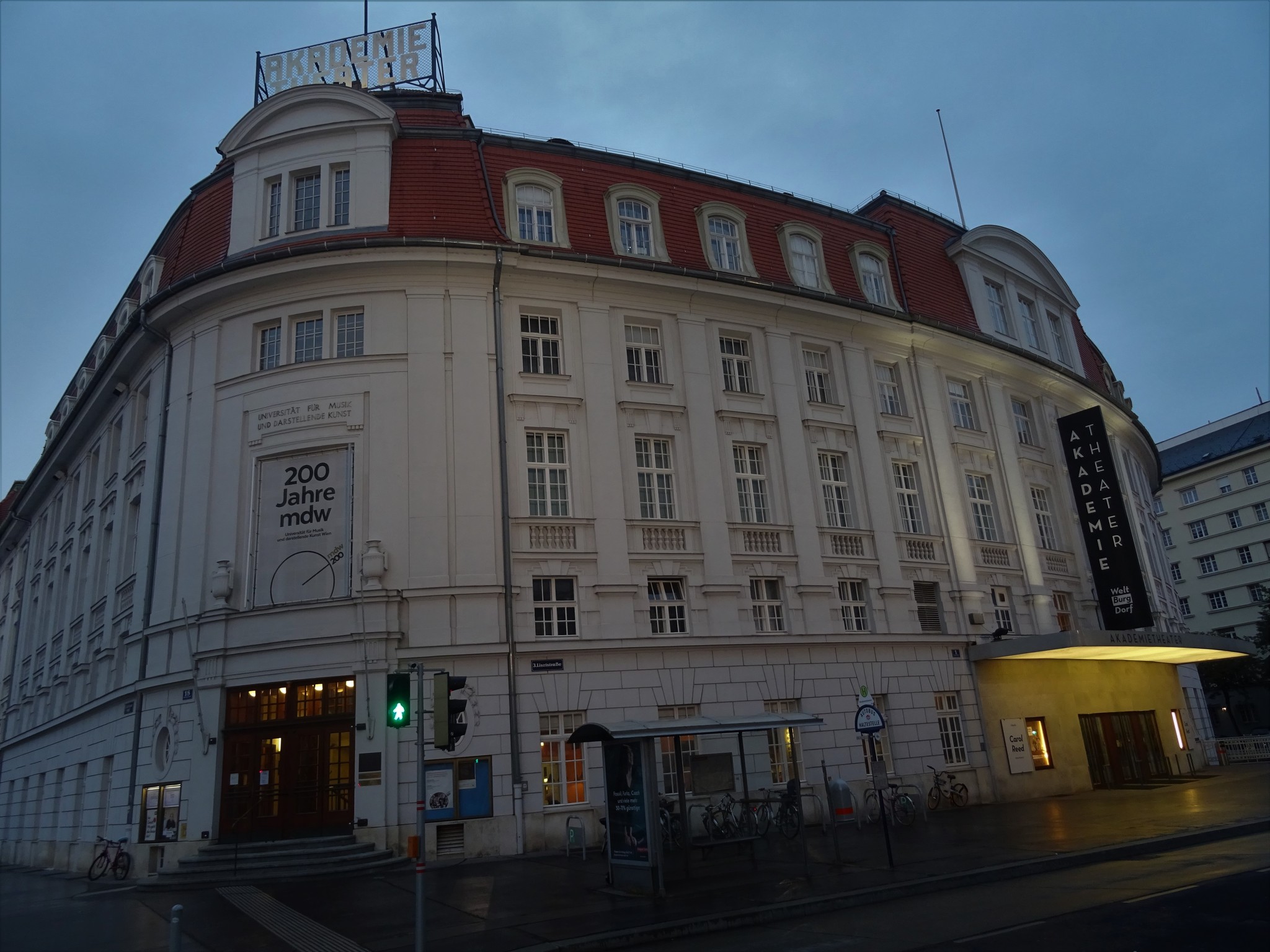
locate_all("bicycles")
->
[658,792,692,851]
[926,764,969,810]
[755,787,800,840]
[865,780,916,826]
[701,792,759,841]
[87,835,132,881]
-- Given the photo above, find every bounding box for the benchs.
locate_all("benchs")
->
[688,834,760,850]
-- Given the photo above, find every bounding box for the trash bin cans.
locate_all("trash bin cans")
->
[830,779,855,822]
[1215,740,1229,766]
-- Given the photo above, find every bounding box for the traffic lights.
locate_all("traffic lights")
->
[386,673,411,730]
[433,671,469,752]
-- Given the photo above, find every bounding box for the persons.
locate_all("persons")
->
[608,744,639,858]
